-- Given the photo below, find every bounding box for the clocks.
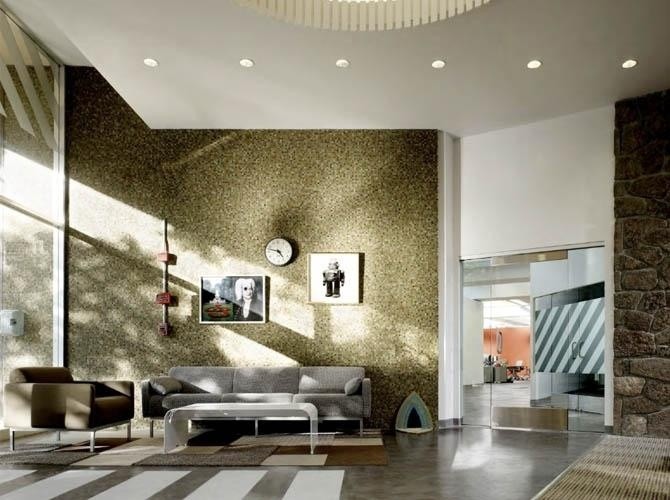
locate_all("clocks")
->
[265,236,293,268]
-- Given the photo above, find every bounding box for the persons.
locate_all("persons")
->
[235,279,263,321]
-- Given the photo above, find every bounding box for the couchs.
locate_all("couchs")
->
[142,366,371,436]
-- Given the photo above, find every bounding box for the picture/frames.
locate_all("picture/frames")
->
[306,251,361,304]
[198,275,267,323]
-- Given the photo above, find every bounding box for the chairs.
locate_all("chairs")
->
[3,367,135,452]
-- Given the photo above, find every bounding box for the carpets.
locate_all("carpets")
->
[134,429,388,468]
[0,428,141,470]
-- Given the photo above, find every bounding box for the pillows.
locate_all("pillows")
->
[149,376,182,396]
[344,377,362,396]
